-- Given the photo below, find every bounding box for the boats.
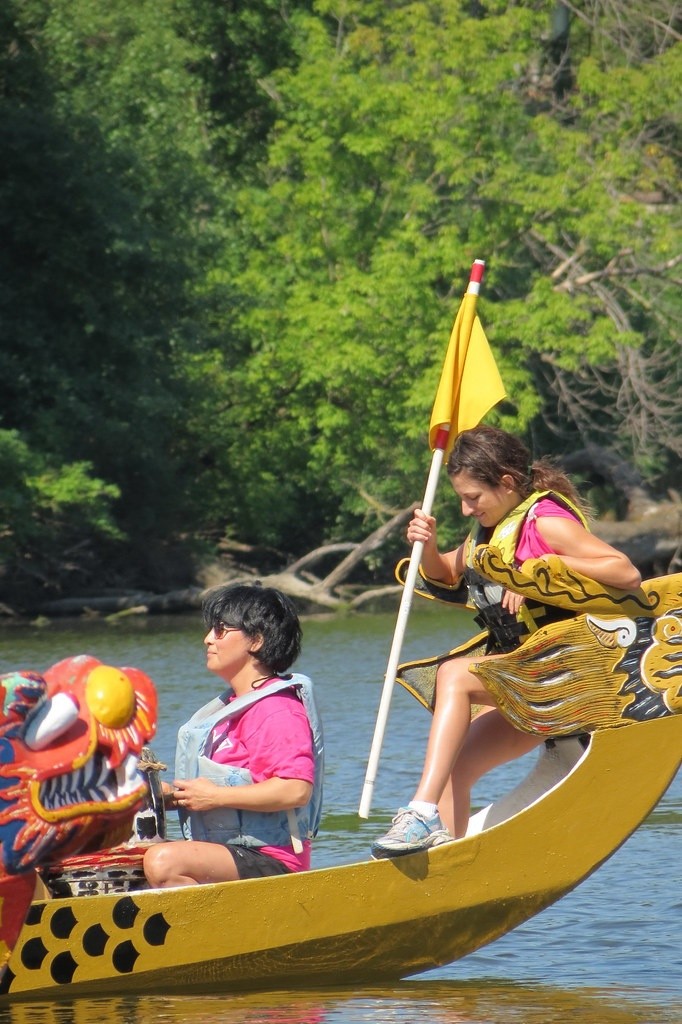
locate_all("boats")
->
[2,655,160,989]
[1,543,681,995]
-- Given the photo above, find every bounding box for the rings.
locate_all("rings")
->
[177,800,186,808]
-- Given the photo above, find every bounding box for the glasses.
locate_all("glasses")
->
[207,622,242,640]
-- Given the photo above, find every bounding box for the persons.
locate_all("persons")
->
[371,430,640,860]
[144,583,326,888]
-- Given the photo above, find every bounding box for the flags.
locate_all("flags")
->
[427,289,506,459]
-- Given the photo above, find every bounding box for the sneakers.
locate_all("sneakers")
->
[371,807,454,859]
